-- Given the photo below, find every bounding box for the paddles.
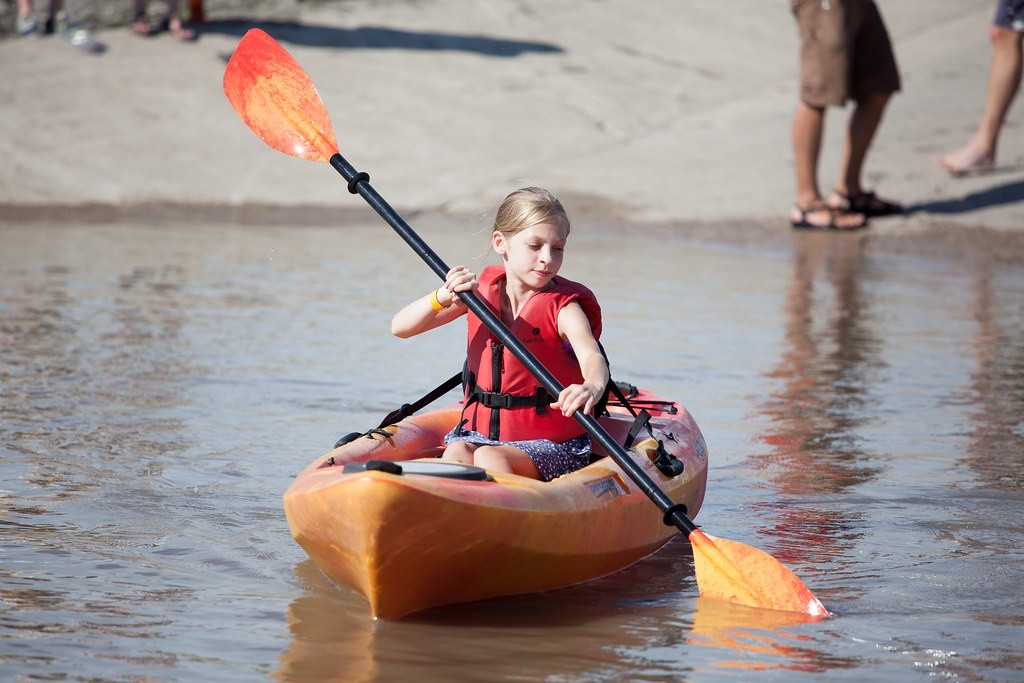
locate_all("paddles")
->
[221,27,830,619]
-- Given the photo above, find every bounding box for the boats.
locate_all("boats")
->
[281,377,709,622]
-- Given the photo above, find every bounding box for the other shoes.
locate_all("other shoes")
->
[15,13,35,35]
[70,31,104,53]
[935,147,999,176]
[170,16,200,43]
[130,12,169,35]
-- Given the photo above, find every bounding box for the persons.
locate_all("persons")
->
[929,0,1024,180]
[391,186,609,475]
[0,0,203,48]
[787,0,904,231]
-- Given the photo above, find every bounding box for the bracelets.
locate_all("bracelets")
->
[430,289,446,313]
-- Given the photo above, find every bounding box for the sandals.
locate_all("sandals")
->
[788,200,868,232]
[827,186,905,218]
